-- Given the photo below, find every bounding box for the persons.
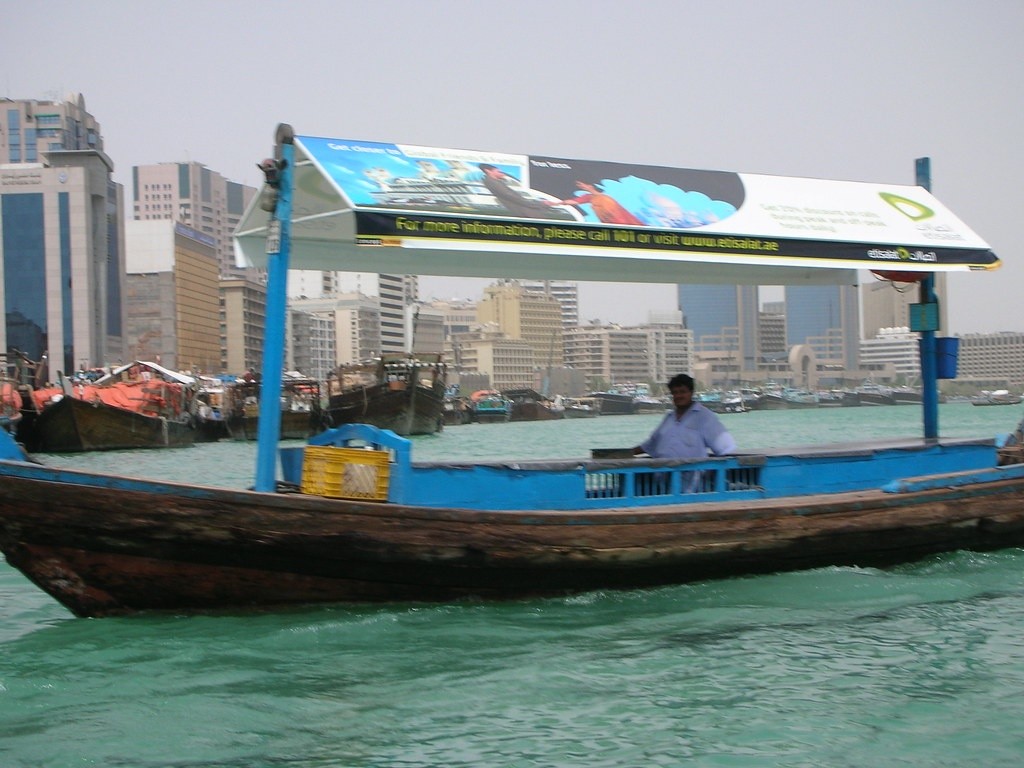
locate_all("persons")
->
[633,373,738,494]
[476,162,643,226]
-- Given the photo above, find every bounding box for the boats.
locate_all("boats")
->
[970,391,1023,406]
[0,122,1024,623]
[221,376,322,442]
[15,359,198,455]
[449,382,946,427]
[328,352,447,437]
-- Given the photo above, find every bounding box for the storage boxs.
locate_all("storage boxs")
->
[300,445,391,502]
